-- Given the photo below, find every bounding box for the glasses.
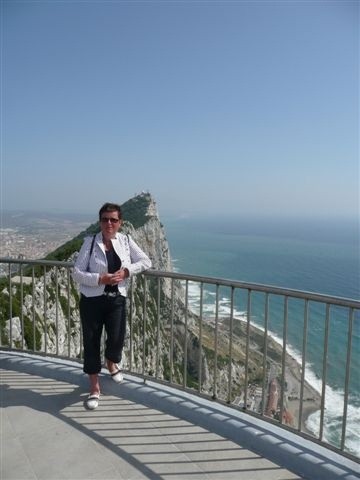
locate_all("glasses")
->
[100,217,119,224]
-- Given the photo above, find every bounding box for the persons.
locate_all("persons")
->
[71,203,151,409]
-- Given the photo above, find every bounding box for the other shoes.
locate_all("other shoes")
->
[110,363,123,383]
[87,390,101,409]
